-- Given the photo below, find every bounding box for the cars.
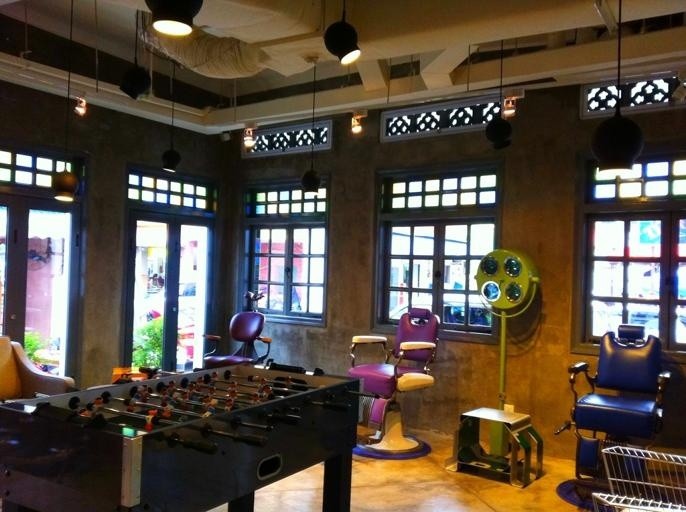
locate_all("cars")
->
[387,295,492,327]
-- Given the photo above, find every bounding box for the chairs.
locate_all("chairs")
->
[345,307,440,460]
[554,323,672,511]
[0,335,77,400]
[200,312,272,367]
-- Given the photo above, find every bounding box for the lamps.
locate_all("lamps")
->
[485,40,513,150]
[323,1,361,65]
[592,1,645,174]
[301,65,320,196]
[51,1,202,203]
